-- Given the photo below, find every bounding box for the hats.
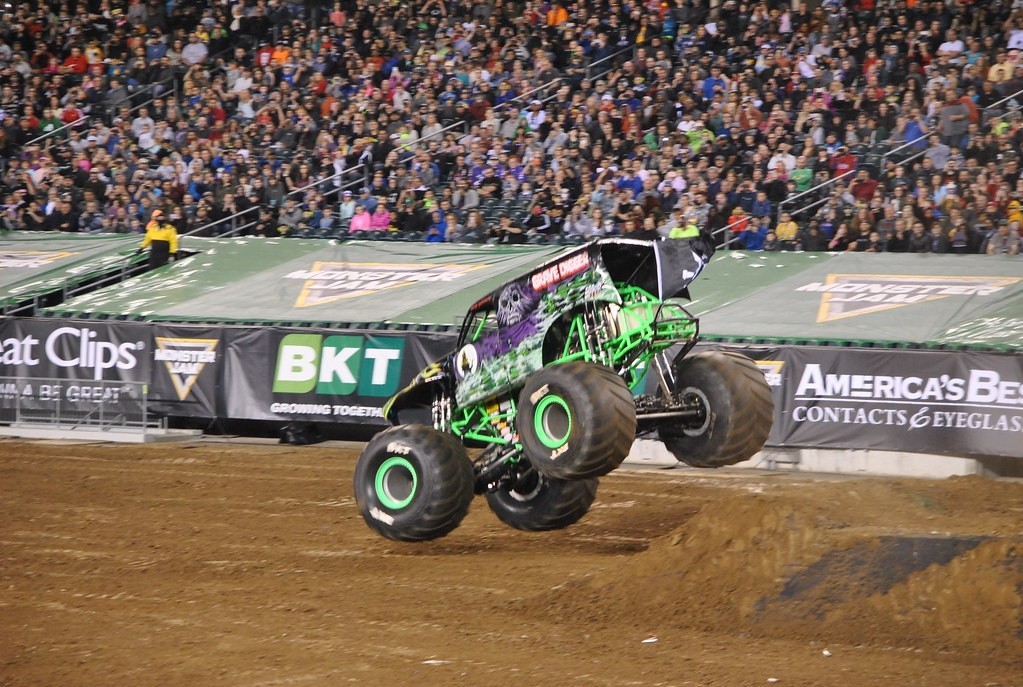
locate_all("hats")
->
[0,0,1023,247]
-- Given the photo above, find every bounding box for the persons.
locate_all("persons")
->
[0,0,1023,256]
[134,206,178,270]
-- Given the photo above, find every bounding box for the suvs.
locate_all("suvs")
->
[353,233,775,542]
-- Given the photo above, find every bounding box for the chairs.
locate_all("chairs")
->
[292,175,589,244]
